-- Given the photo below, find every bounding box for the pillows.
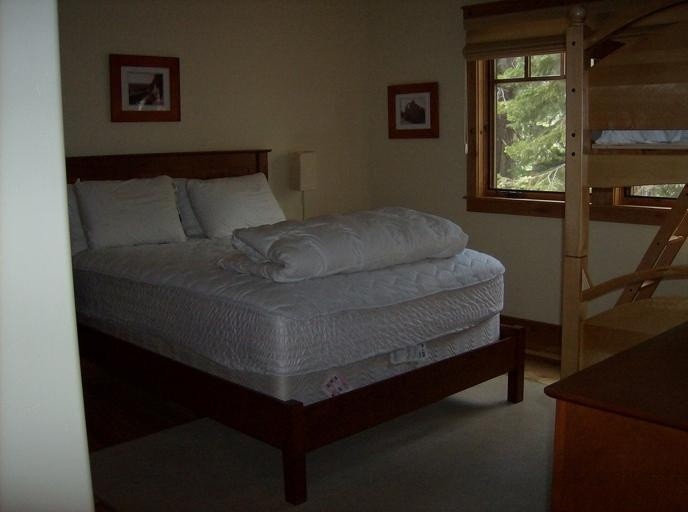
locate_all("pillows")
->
[68,171,287,256]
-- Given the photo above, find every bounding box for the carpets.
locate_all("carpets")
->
[88,374,557,512]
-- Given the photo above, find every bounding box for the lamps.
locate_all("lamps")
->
[291,151,317,220]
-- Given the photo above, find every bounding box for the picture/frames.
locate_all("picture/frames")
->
[108,53,182,123]
[386,81,440,140]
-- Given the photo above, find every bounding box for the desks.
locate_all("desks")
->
[543,321,687,512]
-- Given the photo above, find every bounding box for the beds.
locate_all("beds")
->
[65,148,527,505]
[560,1,688,380]
